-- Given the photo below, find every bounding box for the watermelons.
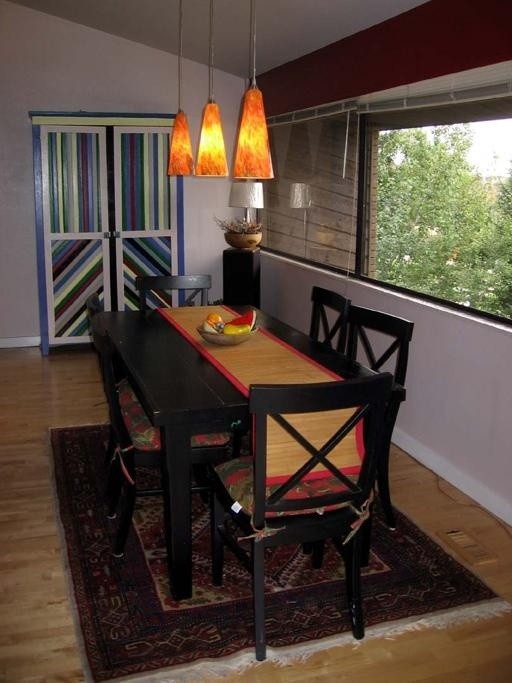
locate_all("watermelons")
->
[225,310,256,330]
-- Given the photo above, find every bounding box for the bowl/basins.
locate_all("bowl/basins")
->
[196,325,260,346]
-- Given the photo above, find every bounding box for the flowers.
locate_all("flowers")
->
[214,215,264,233]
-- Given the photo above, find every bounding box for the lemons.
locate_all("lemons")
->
[224,325,251,334]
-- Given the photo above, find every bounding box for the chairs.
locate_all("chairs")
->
[306,286,415,387]
[209,373,397,661]
[135,275,211,306]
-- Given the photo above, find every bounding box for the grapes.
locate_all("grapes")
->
[216,322,224,329]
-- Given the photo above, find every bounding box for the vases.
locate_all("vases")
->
[225,232,263,251]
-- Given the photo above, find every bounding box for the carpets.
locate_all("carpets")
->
[51,423,511,682]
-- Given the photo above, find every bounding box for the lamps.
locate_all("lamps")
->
[289,183,317,260]
[167,1,275,181]
[227,182,265,225]
